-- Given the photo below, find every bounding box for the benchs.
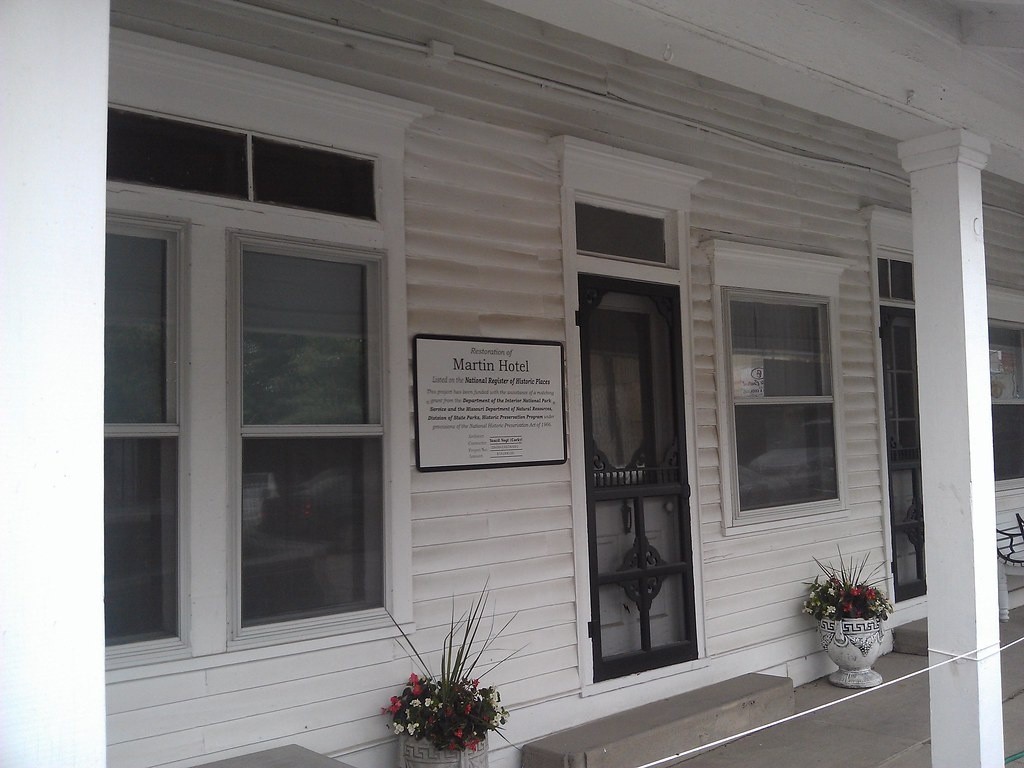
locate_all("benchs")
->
[995,478,1024,622]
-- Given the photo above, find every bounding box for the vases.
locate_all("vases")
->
[400,730,490,768]
[816,617,884,688]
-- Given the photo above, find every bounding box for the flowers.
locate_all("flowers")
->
[378,574,529,752]
[801,543,894,620]
